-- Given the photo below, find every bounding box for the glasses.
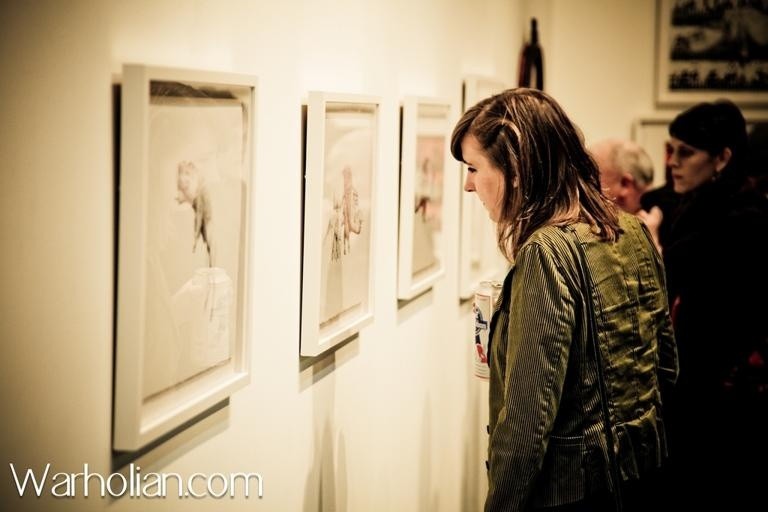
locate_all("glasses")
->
[663,143,696,161]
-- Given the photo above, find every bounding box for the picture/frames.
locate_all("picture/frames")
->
[397,95,459,302]
[631,117,754,191]
[114,65,261,454]
[300,90,384,358]
[459,74,514,300]
[654,0,767,109]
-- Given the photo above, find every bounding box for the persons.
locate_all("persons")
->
[446,85,682,512]
[588,136,652,216]
[635,96,767,511]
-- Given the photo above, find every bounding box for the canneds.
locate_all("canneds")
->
[473,281,503,380]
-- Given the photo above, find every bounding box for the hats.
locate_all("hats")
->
[668,97,750,155]
